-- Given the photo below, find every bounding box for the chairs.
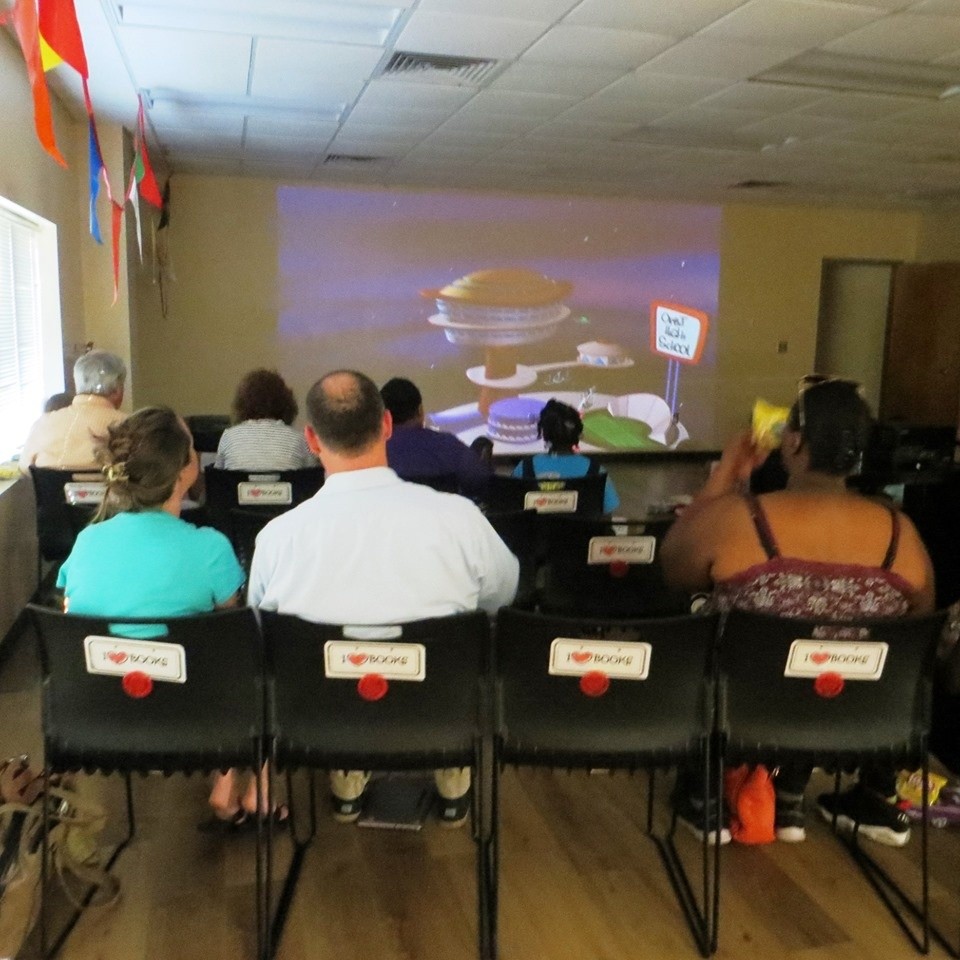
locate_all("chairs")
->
[27,466,949,960]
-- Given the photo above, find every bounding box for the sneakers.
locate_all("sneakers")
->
[439,788,473,828]
[814,790,910,846]
[673,794,732,847]
[775,791,807,842]
[332,790,363,822]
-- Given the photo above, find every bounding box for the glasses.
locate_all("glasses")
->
[798,372,865,428]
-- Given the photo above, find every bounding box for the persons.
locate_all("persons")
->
[376,376,500,511]
[469,436,493,466]
[50,405,294,829]
[210,366,323,475]
[649,370,936,849]
[824,440,959,852]
[245,370,521,827]
[748,375,846,494]
[20,351,147,473]
[513,395,621,517]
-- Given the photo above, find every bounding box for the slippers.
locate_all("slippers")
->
[198,802,294,833]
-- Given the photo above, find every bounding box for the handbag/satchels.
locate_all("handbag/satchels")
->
[724,762,775,843]
[0,785,123,960]
